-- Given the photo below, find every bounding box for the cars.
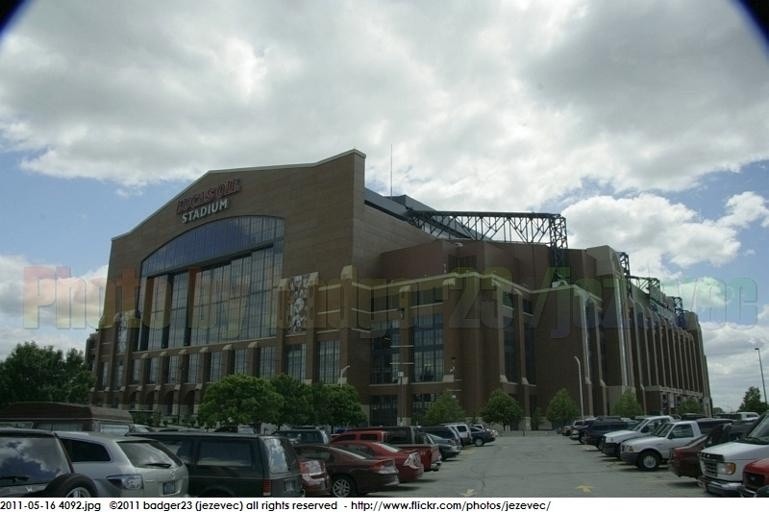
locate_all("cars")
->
[562,411,768,497]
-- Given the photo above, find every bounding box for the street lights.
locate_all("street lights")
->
[754,347,769,409]
[640,383,646,416]
[572,355,585,420]
